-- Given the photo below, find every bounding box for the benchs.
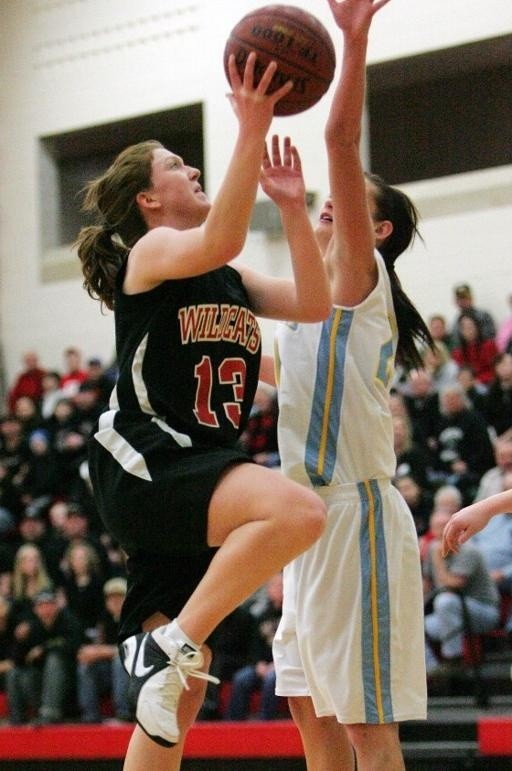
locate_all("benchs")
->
[400,647,512,766]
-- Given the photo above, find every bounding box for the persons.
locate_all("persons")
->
[272,0,441,771]
[1,280,511,731]
[70,48,338,770]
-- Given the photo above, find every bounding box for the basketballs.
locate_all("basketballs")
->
[224,5,335,116]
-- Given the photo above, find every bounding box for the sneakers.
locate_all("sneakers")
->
[116,623,205,749]
